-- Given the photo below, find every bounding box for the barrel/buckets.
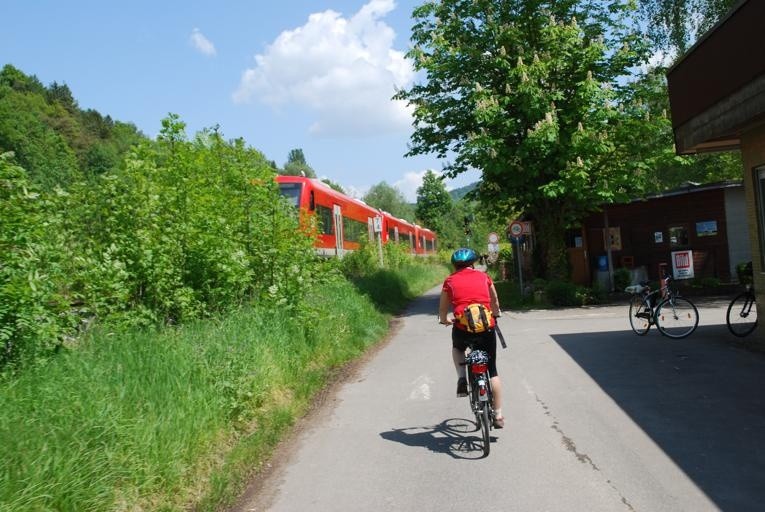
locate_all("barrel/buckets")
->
[597,256,608,270]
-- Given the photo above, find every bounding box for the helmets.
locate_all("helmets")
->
[450,247,481,265]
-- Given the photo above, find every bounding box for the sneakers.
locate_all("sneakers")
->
[456,381,468,397]
[493,416,505,428]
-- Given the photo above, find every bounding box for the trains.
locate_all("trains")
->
[269,175,437,256]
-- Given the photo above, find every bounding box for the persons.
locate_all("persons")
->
[437,247,506,430]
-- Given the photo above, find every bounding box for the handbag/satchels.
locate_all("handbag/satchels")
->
[462,303,492,334]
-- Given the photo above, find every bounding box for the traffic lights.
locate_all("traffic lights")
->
[464,217,473,239]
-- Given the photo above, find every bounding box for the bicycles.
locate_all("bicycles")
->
[439,316,500,457]
[726,262,758,337]
[629,263,699,338]
